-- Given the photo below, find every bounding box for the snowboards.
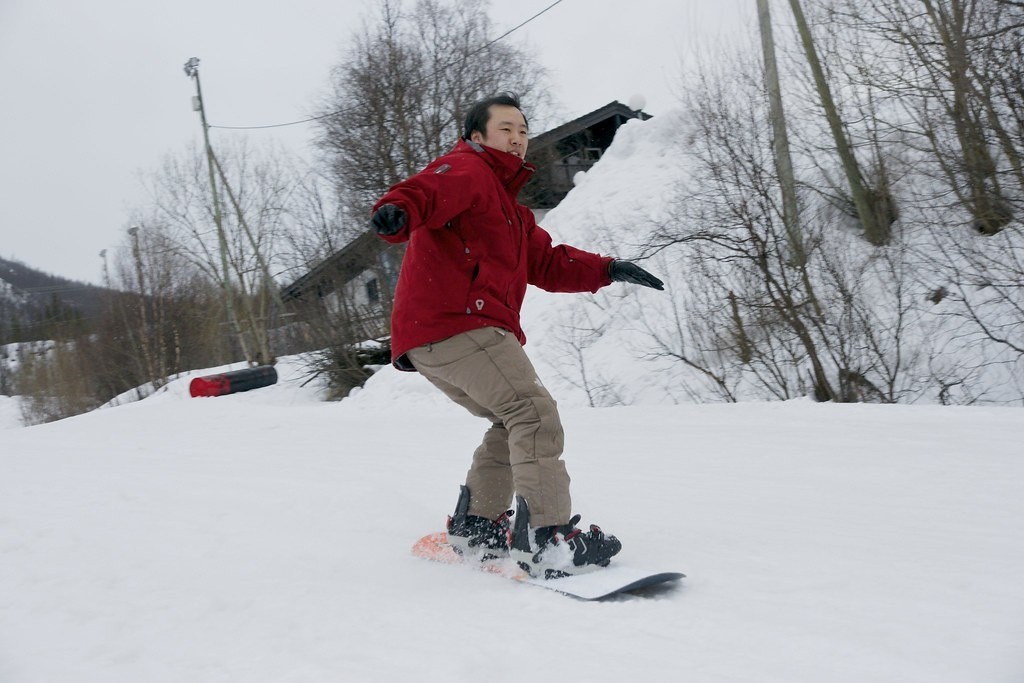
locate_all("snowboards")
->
[406,524,693,611]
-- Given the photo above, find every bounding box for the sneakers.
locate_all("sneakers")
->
[508,495,621,580]
[446,485,514,563]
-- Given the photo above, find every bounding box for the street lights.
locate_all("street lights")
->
[183,55,241,363]
[126,225,148,382]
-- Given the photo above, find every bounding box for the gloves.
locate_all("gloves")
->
[370,204,405,235]
[609,257,665,292]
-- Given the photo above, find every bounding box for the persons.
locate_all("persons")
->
[367,94,666,581]
